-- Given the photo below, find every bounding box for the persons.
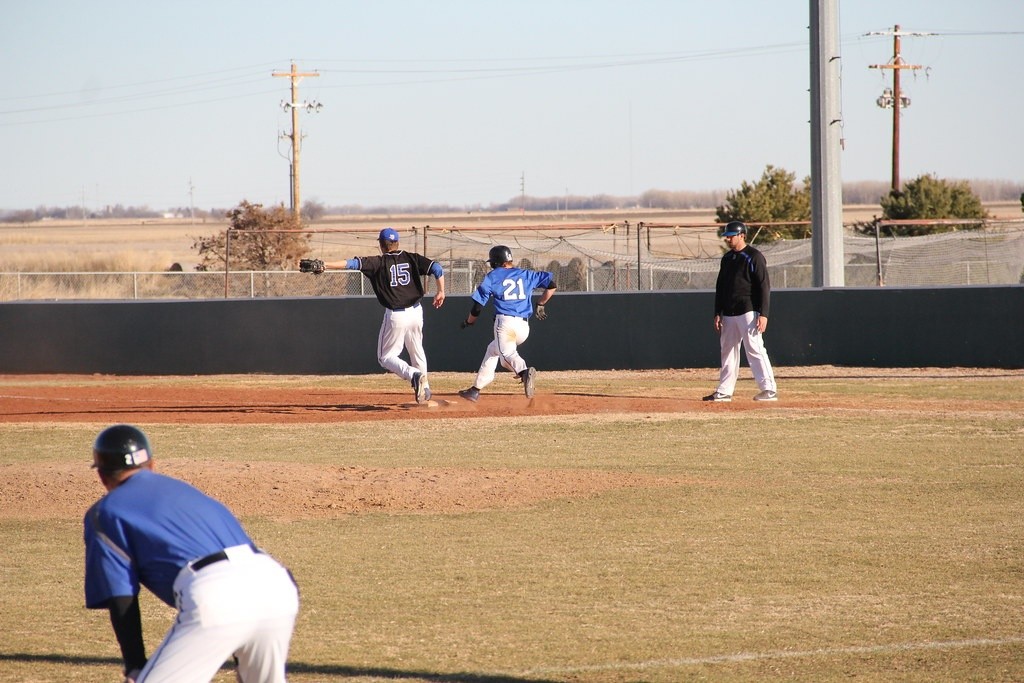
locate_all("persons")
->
[701,221,779,403]
[298,228,445,405]
[458,245,558,403]
[82,425,302,683]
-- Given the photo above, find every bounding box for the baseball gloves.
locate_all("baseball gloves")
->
[299,258,325,275]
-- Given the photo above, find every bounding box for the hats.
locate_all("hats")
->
[377,228,399,241]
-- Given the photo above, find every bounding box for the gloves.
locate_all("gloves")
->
[535,304,548,320]
[461,319,473,330]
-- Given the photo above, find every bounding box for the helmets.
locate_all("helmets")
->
[485,245,513,269]
[92,425,152,469]
[720,221,746,236]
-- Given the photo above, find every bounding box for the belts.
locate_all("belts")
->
[723,313,735,316]
[504,314,527,321]
[393,302,420,312]
[191,543,260,571]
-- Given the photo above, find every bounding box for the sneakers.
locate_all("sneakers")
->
[519,367,536,399]
[411,372,426,405]
[754,390,777,400]
[703,392,732,401]
[424,382,431,401]
[458,386,480,403]
